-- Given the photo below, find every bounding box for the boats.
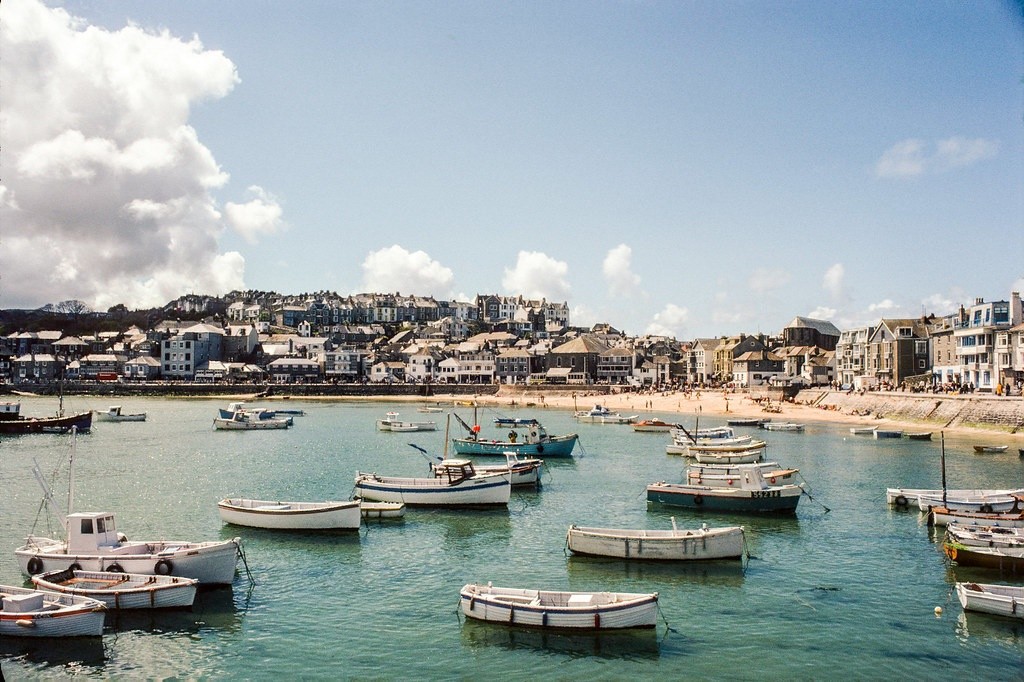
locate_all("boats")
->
[567,517,744,562]
[97,405,146,421]
[885,430,1024,574]
[0,369,93,434]
[417,406,443,413]
[973,445,1009,452]
[451,407,578,459]
[217,499,406,535]
[849,425,934,440]
[574,405,640,423]
[0,425,242,640]
[459,581,658,630]
[353,413,544,511]
[646,415,805,515]
[956,581,1024,621]
[213,402,306,430]
[376,411,436,432]
[630,417,683,431]
[727,416,806,431]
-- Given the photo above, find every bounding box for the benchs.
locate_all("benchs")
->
[257,504,291,511]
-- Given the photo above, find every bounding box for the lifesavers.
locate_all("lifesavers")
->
[979,503,993,513]
[537,445,544,453]
[895,495,909,506]
[154,559,174,575]
[694,495,703,506]
[26,557,42,576]
[106,563,125,572]
[69,563,82,571]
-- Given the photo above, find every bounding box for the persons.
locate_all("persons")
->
[645,399,648,408]
[817,378,842,390]
[847,387,866,395]
[508,430,518,443]
[0,377,491,385]
[633,388,655,395]
[1015,378,1024,390]
[537,395,544,403]
[869,380,906,392]
[762,405,781,414]
[910,381,973,394]
[789,398,842,411]
[568,387,625,399]
[847,410,870,415]
[751,396,771,406]
[997,383,1010,396]
[726,401,728,411]
[875,414,884,419]
[659,387,729,400]
[650,400,652,409]
[700,405,702,413]
